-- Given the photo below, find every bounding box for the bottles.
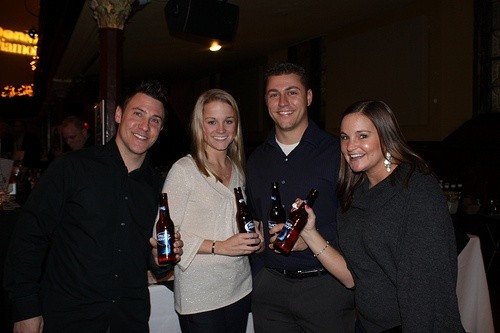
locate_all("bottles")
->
[268,183,286,246]
[156,193,176,265]
[274,188,320,257]
[234,187,258,247]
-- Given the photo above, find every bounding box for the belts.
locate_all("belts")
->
[264,265,326,279]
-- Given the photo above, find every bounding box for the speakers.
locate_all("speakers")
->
[164,0,239,41]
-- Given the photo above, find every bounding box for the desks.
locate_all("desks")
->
[478,208,500,272]
